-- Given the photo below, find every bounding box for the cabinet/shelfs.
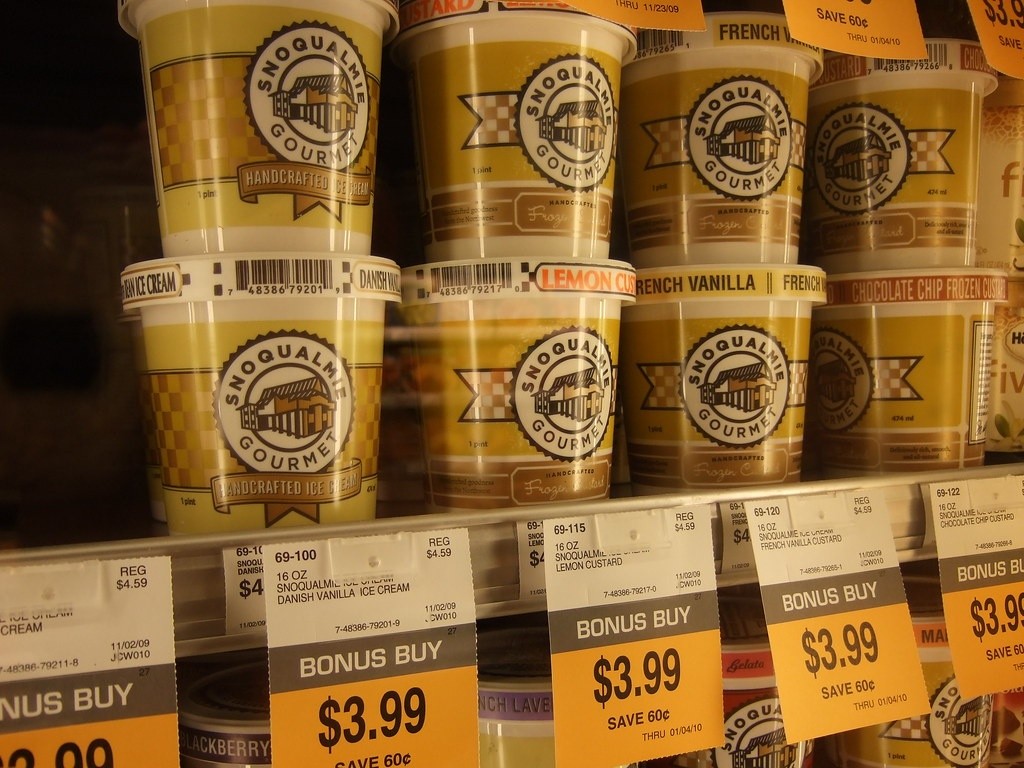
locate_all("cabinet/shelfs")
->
[0,0,1024,768]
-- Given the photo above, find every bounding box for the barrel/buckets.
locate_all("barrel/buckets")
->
[117,1,1022,768]
